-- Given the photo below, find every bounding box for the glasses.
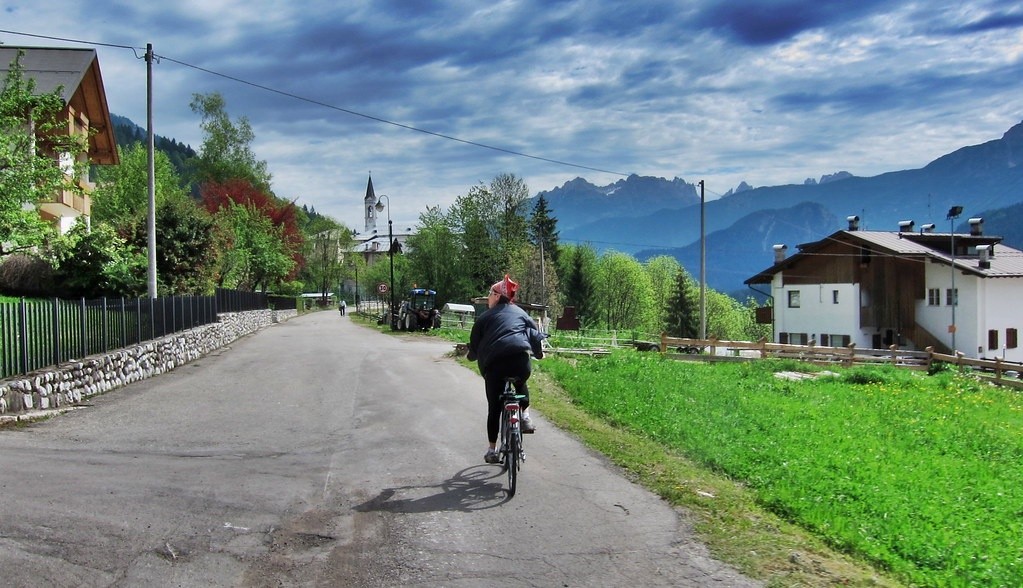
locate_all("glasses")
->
[489,290,499,295]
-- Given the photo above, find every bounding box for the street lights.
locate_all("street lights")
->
[947,205,963,358]
[351,263,359,312]
[374,194,398,332]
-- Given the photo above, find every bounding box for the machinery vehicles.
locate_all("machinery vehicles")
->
[390,287,442,333]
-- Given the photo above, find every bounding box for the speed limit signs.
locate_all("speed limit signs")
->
[378,283,387,292]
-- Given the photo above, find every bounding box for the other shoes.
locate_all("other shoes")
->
[485,451,499,463]
[521,416,533,430]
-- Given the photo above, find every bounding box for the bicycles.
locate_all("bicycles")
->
[486,353,536,498]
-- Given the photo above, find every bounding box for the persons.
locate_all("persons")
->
[340,298,346,316]
[467,275,544,462]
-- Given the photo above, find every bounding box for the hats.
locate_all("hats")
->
[492,275,518,300]
